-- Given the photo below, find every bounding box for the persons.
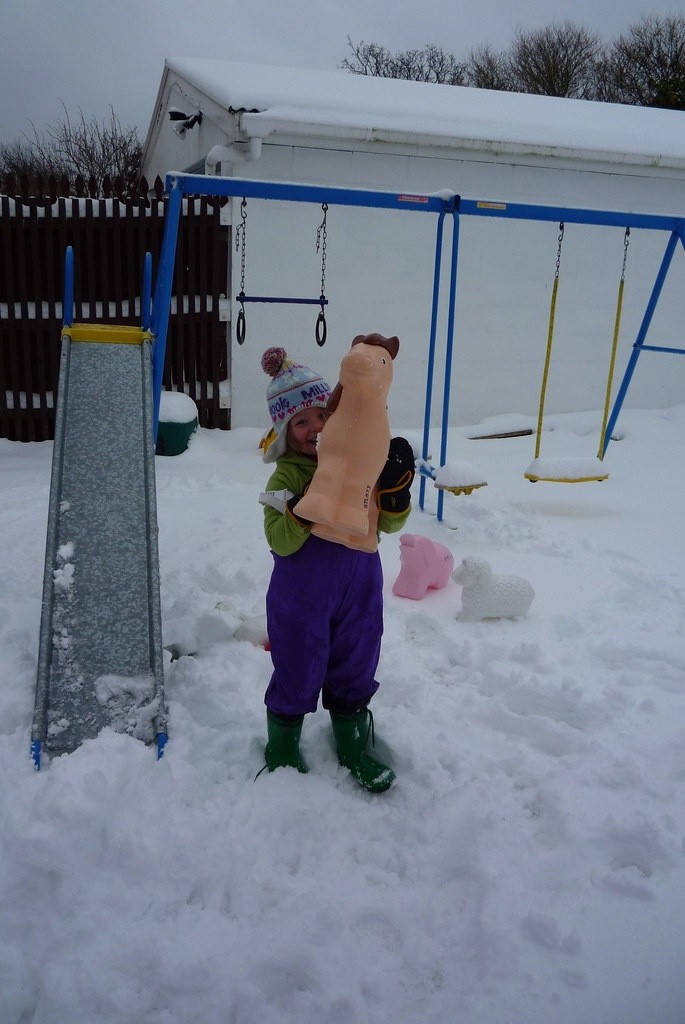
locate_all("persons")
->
[261,346,415,794]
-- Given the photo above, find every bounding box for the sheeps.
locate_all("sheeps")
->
[451,556,535,624]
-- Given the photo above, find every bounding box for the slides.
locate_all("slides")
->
[29,322,168,770]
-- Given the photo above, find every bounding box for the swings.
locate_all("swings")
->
[523,235,628,483]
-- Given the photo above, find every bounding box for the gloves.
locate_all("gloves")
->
[284,478,315,528]
[375,436,413,513]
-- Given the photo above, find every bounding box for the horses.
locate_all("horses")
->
[392,533,454,600]
[292,331,402,554]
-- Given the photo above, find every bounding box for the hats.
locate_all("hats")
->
[260,346,334,464]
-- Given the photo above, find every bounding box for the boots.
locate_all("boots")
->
[331,707,395,792]
[263,704,306,774]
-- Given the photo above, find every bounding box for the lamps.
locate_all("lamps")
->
[169,111,203,140]
